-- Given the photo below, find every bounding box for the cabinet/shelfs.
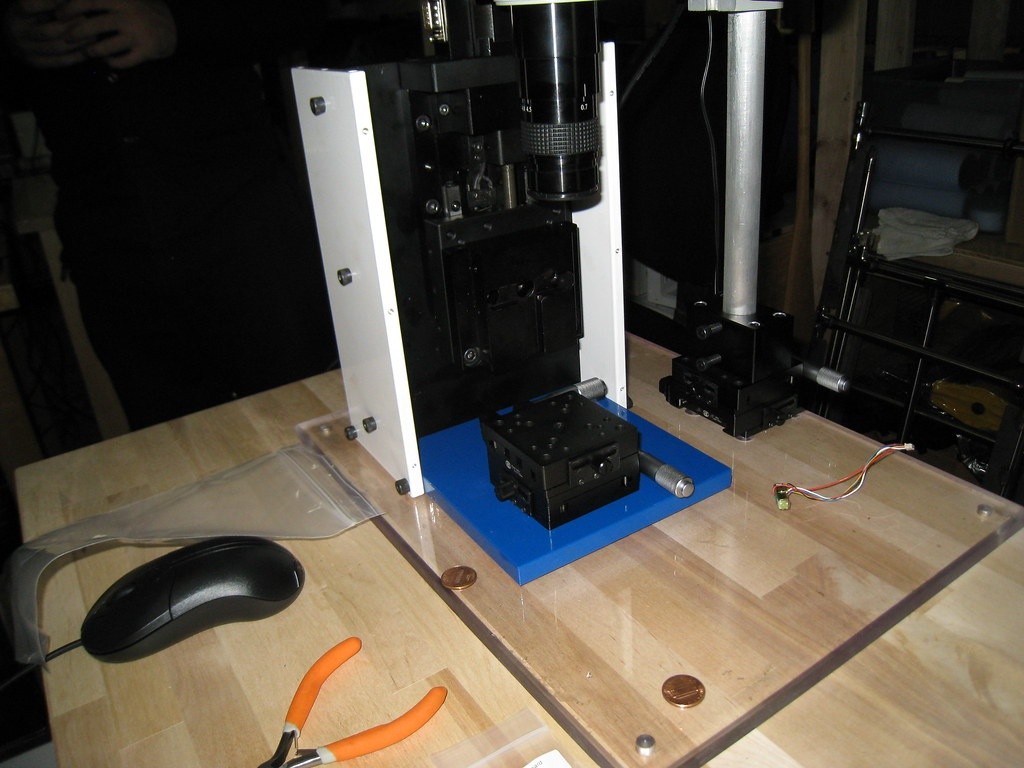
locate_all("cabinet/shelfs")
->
[787,58,1024,501]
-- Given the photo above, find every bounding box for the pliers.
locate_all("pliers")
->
[255,637,449,768]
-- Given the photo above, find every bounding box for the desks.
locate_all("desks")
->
[10,365,1024,768]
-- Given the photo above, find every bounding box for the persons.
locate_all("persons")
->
[6,0,338,430]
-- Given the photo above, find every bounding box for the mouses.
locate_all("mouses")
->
[80,537,305,663]
